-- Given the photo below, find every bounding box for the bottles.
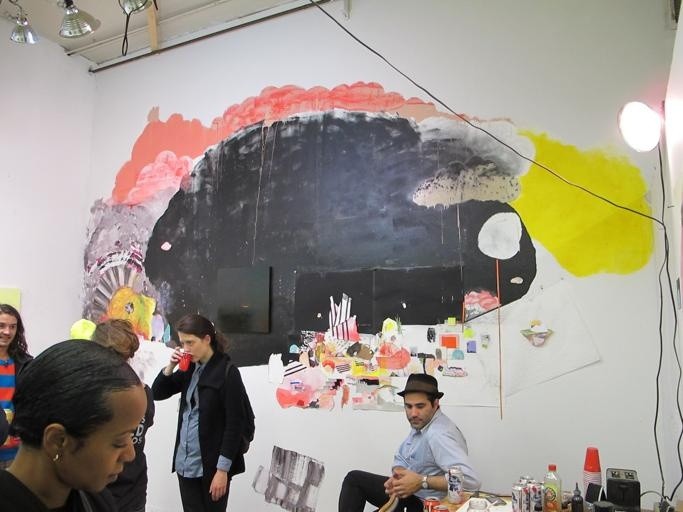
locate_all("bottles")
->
[543,463,562,512]
[571,482,583,511]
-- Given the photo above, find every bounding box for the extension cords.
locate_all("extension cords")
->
[654,500,666,511]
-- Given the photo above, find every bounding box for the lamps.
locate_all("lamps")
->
[57,0,101,38]
[8,0,39,45]
[123,0,153,14]
[619,100,666,154]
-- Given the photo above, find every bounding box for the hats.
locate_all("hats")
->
[397,373,444,399]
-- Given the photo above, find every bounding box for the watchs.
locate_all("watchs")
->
[422,473,429,489]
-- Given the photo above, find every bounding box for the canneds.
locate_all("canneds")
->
[447,465,462,504]
[422,495,440,512]
[519,474,534,485]
[528,479,543,511]
[431,505,449,512]
[512,484,528,512]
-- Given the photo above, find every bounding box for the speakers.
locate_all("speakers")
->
[659,499,675,512]
[584,482,605,505]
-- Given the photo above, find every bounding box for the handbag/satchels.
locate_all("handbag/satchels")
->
[234,376,255,458]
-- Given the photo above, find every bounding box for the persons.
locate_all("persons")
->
[337,372,479,512]
[68,318,153,512]
[151,313,254,512]
[0,339,147,511]
[0,305,35,471]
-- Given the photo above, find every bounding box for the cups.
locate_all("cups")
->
[177,351,193,371]
[583,446,601,500]
